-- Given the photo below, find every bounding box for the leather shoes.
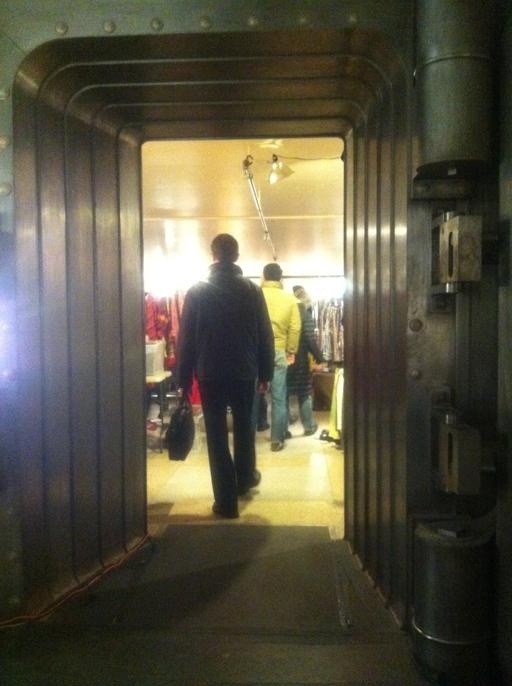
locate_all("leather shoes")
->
[212,470,261,519]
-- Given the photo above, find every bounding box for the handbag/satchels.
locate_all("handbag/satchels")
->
[168,401,195,461]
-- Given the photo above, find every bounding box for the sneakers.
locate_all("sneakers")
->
[257,414,317,451]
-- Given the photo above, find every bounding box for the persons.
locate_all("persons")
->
[282,285,327,445]
[173,233,271,520]
[255,263,302,454]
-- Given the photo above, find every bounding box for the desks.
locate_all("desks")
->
[146,372,172,454]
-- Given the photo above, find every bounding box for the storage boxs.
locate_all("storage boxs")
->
[146,338,166,376]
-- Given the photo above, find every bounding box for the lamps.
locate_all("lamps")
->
[265,153,294,185]
